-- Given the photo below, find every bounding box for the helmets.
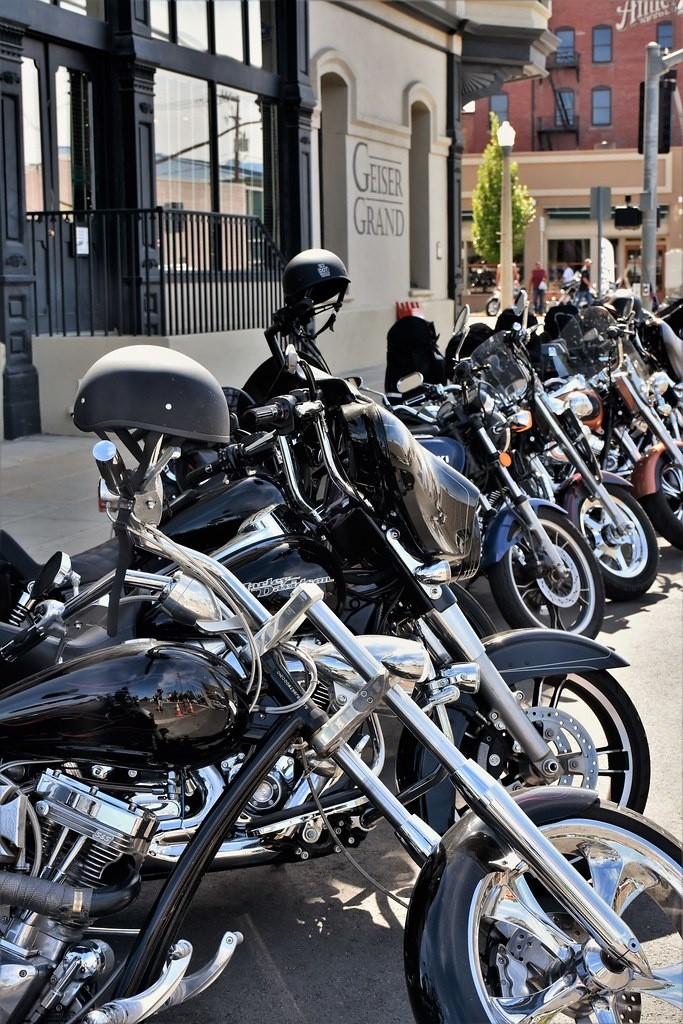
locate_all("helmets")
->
[73,345,231,444]
[283,249,351,305]
[603,289,642,323]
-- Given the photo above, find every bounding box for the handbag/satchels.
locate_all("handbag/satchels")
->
[538,281,547,290]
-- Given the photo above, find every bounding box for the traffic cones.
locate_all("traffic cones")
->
[175,703,184,717]
[189,702,195,713]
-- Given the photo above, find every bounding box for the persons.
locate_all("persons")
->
[496,258,593,316]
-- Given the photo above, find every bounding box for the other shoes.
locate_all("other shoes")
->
[533,312,544,317]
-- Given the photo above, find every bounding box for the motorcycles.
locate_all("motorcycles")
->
[0,248,683,1024]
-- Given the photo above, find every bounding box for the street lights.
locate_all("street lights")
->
[496,120,516,314]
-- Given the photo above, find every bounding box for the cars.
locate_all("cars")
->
[211,700,227,710]
